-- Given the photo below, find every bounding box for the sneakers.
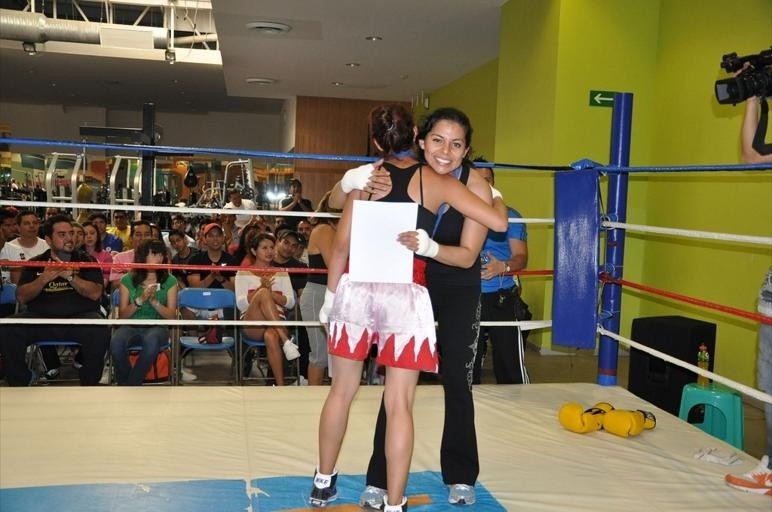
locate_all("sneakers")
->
[39,368,61,384]
[446,484,476,507]
[358,484,408,512]
[180,358,198,383]
[99,360,114,386]
[724,454,771,496]
[307,465,340,509]
[281,337,302,361]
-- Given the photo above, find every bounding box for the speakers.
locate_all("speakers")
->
[629,317,715,422]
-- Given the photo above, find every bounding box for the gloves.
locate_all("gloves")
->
[557,401,615,434]
[604,409,657,438]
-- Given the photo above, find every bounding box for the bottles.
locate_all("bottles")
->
[696,341,709,386]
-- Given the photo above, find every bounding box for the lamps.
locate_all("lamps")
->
[22,1,38,55]
[166,1,177,63]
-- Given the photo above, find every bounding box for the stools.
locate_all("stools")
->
[680,383,742,455]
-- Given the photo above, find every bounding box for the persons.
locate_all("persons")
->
[725,58,772,496]
[307,105,508,512]
[0,157,531,386]
[328,107,494,509]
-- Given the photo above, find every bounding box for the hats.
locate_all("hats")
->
[204,223,223,237]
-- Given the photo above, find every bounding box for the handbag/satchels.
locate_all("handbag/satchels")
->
[197,314,223,345]
